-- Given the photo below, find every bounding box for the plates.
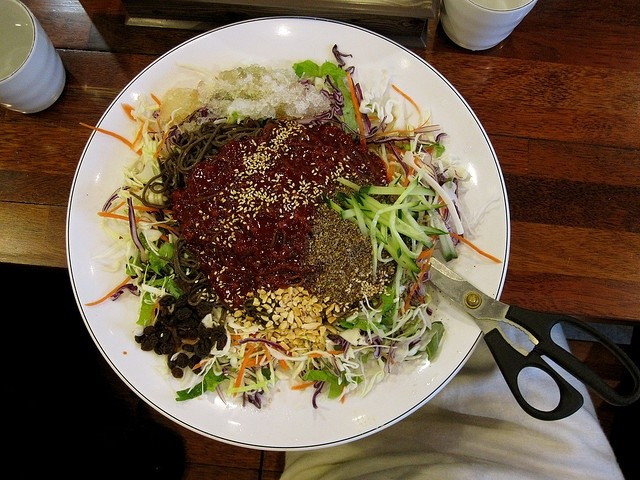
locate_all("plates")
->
[62,12,513,453]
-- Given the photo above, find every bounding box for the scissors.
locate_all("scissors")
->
[426,256,640,420]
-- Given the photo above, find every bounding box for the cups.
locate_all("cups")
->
[446,0,537,50]
[0,0,68,115]
